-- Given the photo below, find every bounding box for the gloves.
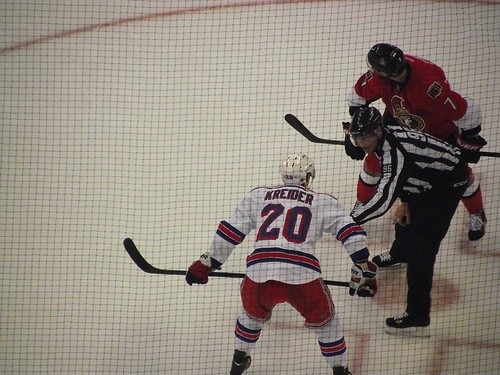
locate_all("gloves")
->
[342,121,366,160]
[349,260,377,298]
[455,133,487,164]
[186,251,223,286]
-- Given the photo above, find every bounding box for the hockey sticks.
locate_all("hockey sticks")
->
[123,238,370,291]
[284,113,500,158]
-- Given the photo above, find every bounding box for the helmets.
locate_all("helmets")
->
[366,43,408,83]
[279,154,315,186]
[350,107,383,137]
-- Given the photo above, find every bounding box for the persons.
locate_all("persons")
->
[349,107,468,334]
[187,153,376,375]
[345,43,487,243]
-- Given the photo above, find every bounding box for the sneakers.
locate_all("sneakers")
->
[468,210,487,247]
[385,312,430,336]
[333,366,352,375]
[372,251,400,267]
[230,349,251,375]
